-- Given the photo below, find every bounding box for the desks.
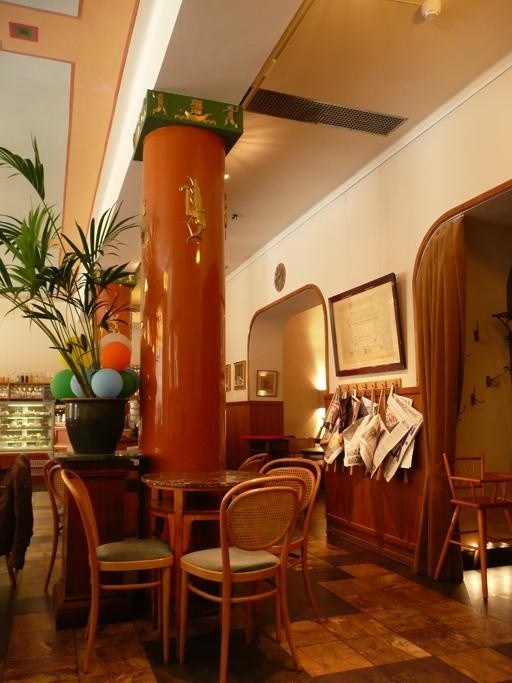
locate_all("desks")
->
[240,436,295,453]
[139,471,268,666]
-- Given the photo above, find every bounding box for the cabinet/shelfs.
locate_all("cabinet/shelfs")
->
[0,383,55,450]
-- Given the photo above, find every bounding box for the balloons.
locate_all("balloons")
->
[97,341,132,373]
[99,330,133,354]
[56,335,97,370]
[69,369,93,397]
[49,368,73,400]
[116,369,141,399]
[90,367,124,399]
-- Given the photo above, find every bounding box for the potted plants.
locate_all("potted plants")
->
[0,129,140,458]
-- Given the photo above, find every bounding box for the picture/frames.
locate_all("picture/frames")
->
[328,272,406,377]
[225,360,277,397]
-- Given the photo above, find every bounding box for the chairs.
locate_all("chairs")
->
[229,453,268,504]
[43,456,66,594]
[0,453,25,587]
[257,457,323,643]
[59,468,175,675]
[177,473,309,683]
[432,451,512,602]
